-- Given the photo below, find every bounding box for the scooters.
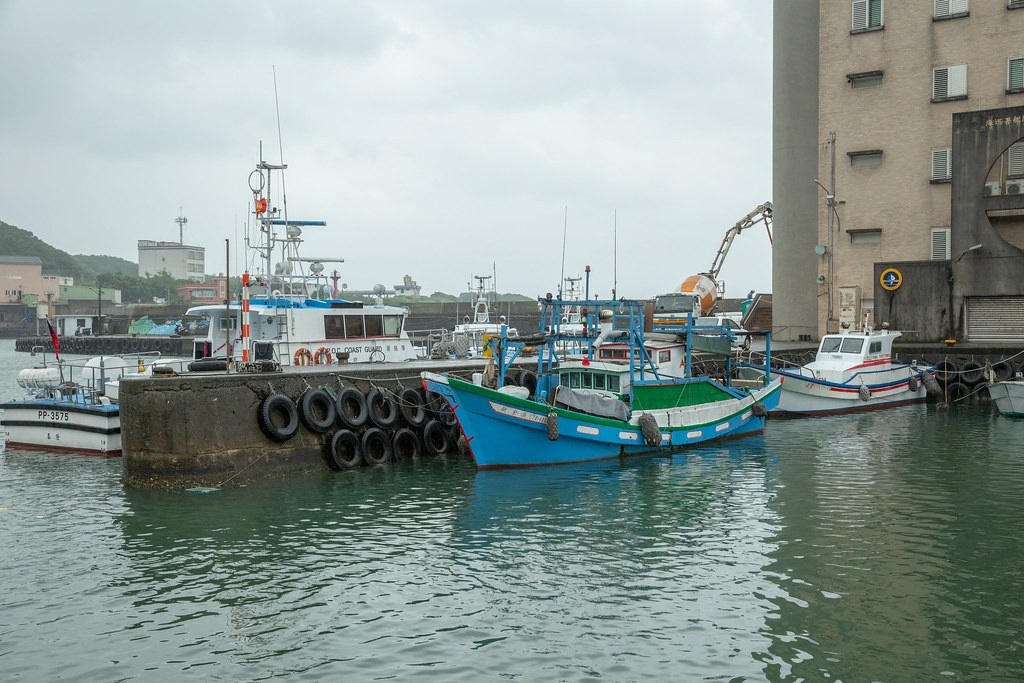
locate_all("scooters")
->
[75,326,90,336]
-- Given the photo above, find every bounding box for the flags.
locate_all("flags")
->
[46,318,60,361]
[254,196,267,219]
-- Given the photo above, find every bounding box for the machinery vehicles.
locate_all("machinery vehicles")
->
[652,271,722,344]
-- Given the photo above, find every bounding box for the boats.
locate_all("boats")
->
[1,140,470,460]
[986,378,1024,418]
[735,313,938,423]
[430,263,614,358]
[415,263,785,475]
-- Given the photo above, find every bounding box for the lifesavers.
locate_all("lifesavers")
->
[271,290,281,298]
[315,347,332,365]
[294,348,314,365]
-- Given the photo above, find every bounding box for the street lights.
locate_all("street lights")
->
[88,286,104,330]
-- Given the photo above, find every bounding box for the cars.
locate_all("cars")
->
[693,316,753,352]
